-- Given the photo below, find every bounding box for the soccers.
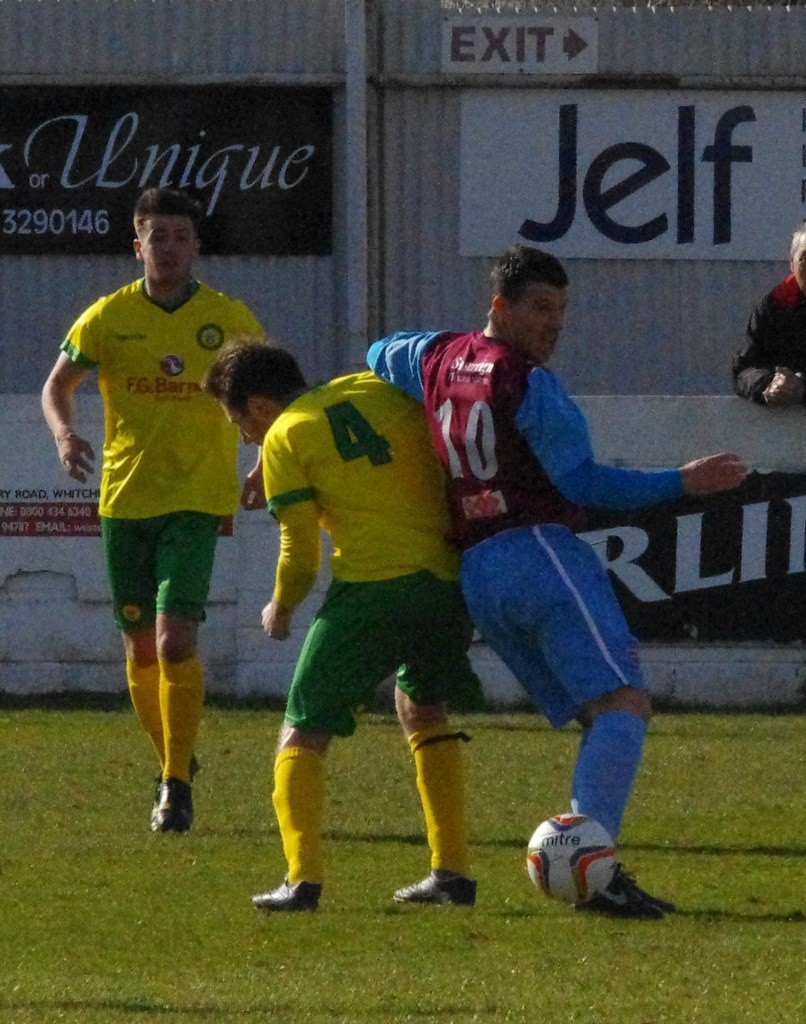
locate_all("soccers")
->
[525,813,616,905]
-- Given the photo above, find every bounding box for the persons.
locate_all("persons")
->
[368,248,748,916]
[731,226,806,404]
[41,192,268,831]
[205,343,476,906]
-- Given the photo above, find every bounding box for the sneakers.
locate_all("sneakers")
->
[574,863,663,919]
[393,868,478,907]
[150,777,193,834]
[251,878,322,912]
[154,752,200,803]
[627,878,676,913]
